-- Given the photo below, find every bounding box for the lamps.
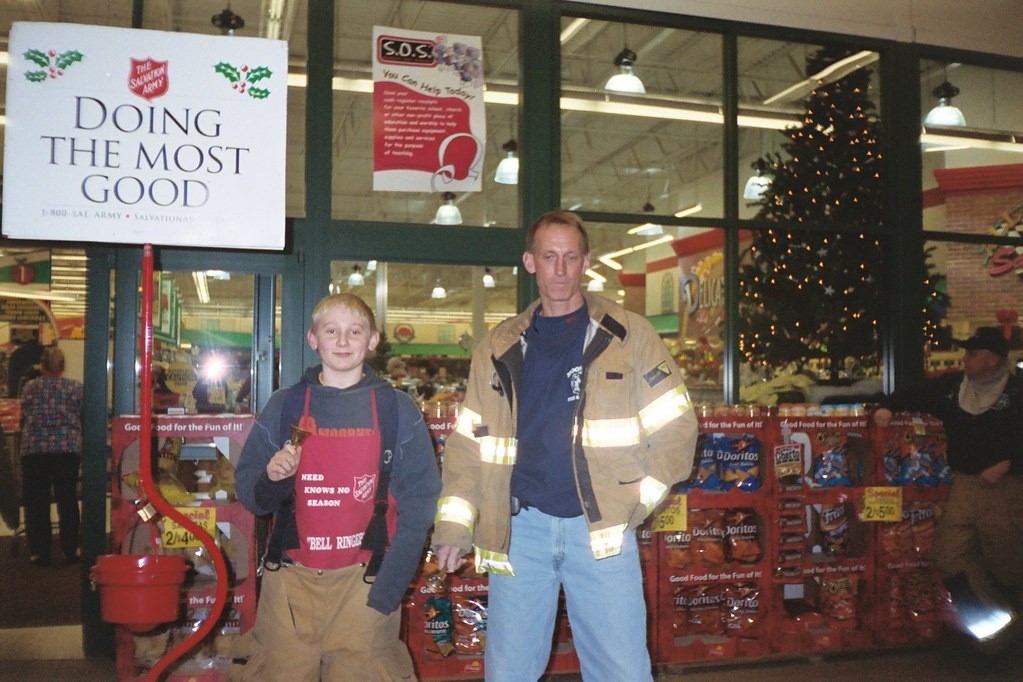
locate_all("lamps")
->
[483,267,496,287]
[431,278,446,299]
[190,271,210,303]
[208,269,230,280]
[640,202,663,234]
[436,192,463,225]
[586,279,604,292]
[494,140,519,185]
[604,22,646,93]
[925,63,966,126]
[260,1,293,40]
[559,18,588,44]
[743,128,774,200]
[348,265,364,285]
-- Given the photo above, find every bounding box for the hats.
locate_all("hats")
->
[953,327,1010,357]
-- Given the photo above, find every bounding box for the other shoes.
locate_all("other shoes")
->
[29,553,50,565]
[63,552,80,564]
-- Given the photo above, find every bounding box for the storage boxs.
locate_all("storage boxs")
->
[110,413,954,682]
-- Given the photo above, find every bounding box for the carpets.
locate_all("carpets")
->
[0,534,107,630]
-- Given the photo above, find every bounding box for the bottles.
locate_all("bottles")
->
[768,403,869,416]
[417,402,429,418]
[433,402,448,416]
[694,403,764,417]
[449,402,459,418]
[889,411,942,426]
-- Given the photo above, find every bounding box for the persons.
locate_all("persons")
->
[18,348,84,563]
[192,366,279,414]
[873,326,1023,660]
[431,213,700,682]
[234,293,443,682]
